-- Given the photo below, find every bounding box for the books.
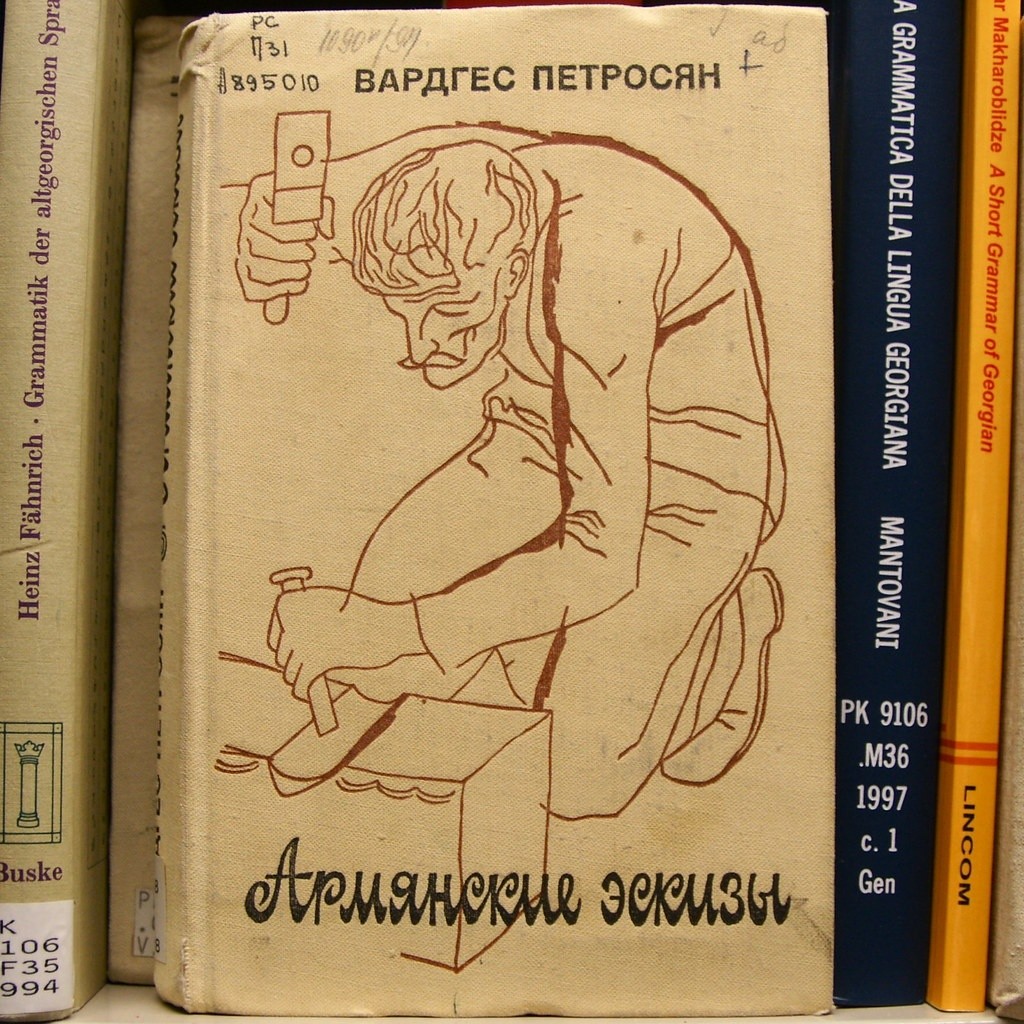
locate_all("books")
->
[0,0,1024,1024]
